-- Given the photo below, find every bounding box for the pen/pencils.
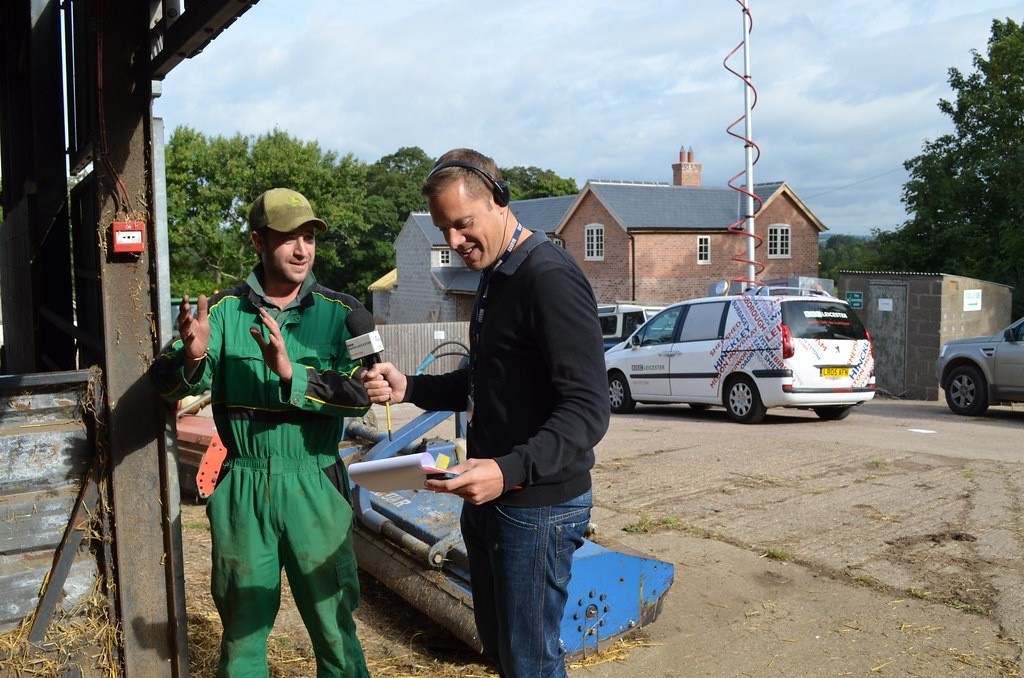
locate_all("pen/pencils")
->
[386,402,392,442]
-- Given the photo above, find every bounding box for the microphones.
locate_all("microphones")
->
[343,309,391,401]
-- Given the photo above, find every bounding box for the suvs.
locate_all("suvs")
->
[936,317,1024,417]
[606,295,874,425]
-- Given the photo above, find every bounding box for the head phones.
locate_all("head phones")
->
[427,160,510,208]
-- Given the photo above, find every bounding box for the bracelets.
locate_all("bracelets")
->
[185,347,210,361]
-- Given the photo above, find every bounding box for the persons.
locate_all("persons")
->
[359,149,611,677]
[147,188,376,678]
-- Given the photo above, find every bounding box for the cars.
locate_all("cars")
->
[596,305,679,353]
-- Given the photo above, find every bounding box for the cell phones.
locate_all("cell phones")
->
[426,471,460,480]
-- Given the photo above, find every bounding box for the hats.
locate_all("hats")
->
[249,187,328,232]
